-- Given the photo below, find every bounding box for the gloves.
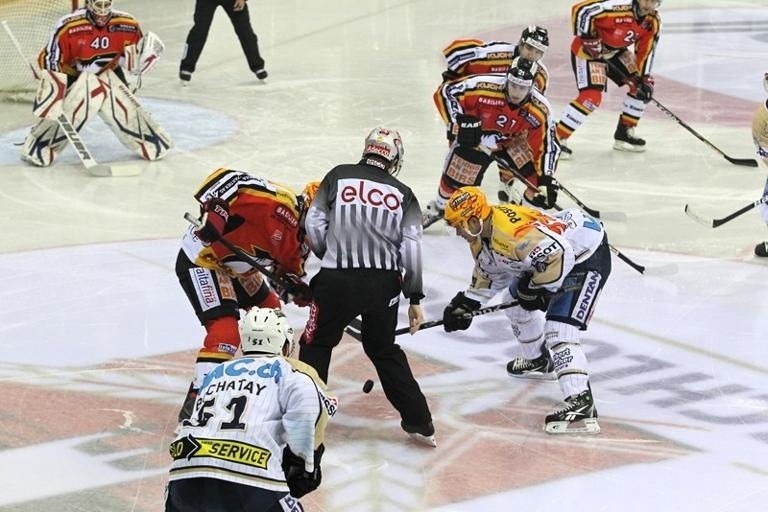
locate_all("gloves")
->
[291,283,310,307]
[445,118,483,147]
[532,175,558,210]
[444,292,482,333]
[518,271,543,303]
[193,198,228,244]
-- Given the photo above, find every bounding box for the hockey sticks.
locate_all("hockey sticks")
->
[453,124,679,277]
[184,211,363,344]
[2,21,141,177]
[583,45,763,168]
[558,140,573,157]
[684,197,766,230]
[552,178,627,223]
[343,301,519,343]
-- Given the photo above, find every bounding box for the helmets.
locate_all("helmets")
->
[240,307,295,358]
[441,186,492,237]
[506,0,660,94]
[363,126,403,177]
[301,181,320,208]
[84,1,112,28]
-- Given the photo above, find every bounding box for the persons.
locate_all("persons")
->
[297,124,438,439]
[179,0,269,84]
[175,167,326,424]
[749,72,768,257]
[441,22,553,207]
[22,0,165,165]
[162,302,337,512]
[443,183,613,426]
[550,0,663,157]
[420,54,565,231]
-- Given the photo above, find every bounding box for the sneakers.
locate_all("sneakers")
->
[401,419,434,436]
[561,139,571,153]
[613,125,646,146]
[255,69,265,81]
[179,69,192,85]
[547,391,598,422]
[505,359,555,374]
[422,201,442,229]
